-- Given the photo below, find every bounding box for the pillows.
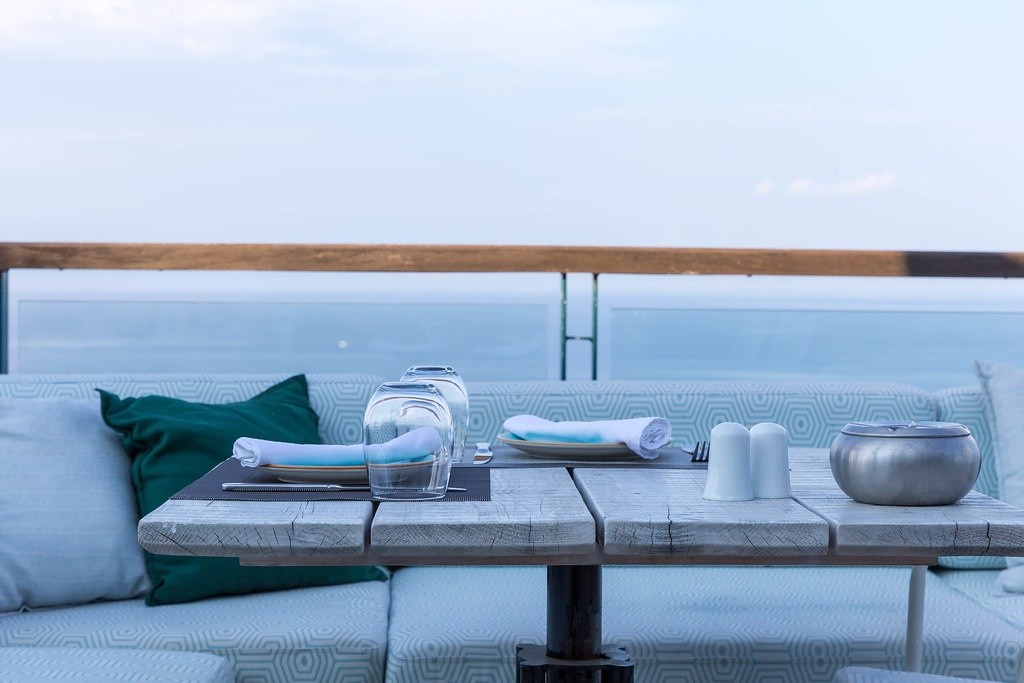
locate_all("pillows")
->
[0,397,153,615]
[95,373,393,606]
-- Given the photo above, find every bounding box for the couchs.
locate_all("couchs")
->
[0,372,1024,683]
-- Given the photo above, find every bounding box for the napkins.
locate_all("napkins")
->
[502,414,675,461]
[232,426,440,468]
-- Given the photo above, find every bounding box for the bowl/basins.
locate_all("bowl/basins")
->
[829,419,982,504]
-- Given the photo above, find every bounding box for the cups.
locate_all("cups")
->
[363,381,454,501]
[702,421,753,500]
[749,422,792,499]
[401,365,470,463]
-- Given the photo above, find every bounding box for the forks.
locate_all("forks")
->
[681,441,710,462]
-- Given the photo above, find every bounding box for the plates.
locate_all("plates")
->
[497,433,673,459]
[259,460,432,484]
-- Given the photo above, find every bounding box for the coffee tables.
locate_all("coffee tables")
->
[135,447,1024,683]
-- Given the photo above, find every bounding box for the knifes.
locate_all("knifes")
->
[222,482,467,492]
[473,443,494,465]
